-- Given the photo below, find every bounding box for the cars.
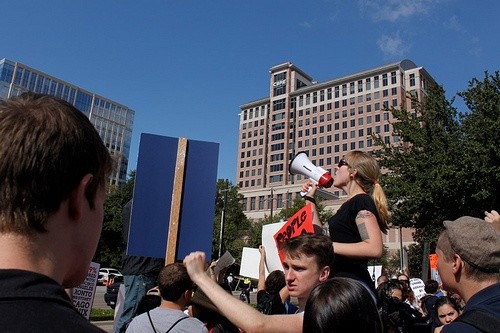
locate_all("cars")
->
[97,268,123,285]
[104,280,124,309]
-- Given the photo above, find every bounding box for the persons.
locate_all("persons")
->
[184,233,334,333]
[137,271,299,333]
[125,264,208,333]
[435,210,500,333]
[301,150,390,292]
[302,277,382,333]
[377,266,462,333]
[0,91,112,333]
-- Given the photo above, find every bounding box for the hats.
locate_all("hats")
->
[443,217,500,272]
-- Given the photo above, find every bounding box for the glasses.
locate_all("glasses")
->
[338,159,352,169]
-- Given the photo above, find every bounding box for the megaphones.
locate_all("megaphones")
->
[289,150,334,197]
